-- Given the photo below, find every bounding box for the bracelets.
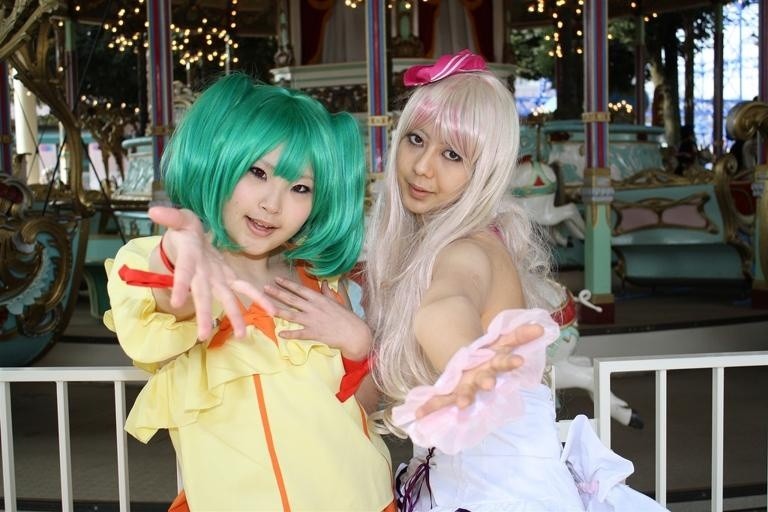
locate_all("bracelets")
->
[341,351,377,376]
[160,238,175,274]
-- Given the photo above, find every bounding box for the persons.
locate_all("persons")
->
[103,74,397,512]
[366,71,585,512]
